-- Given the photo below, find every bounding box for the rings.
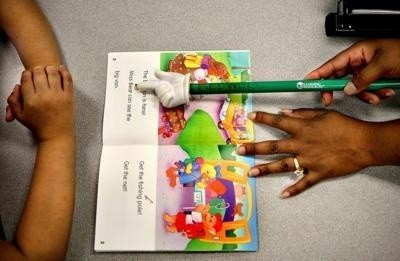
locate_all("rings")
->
[293,157,304,176]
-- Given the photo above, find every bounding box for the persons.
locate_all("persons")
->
[237,37,400,198]
[1,0,76,260]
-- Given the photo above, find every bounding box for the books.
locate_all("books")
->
[94,49,261,252]
